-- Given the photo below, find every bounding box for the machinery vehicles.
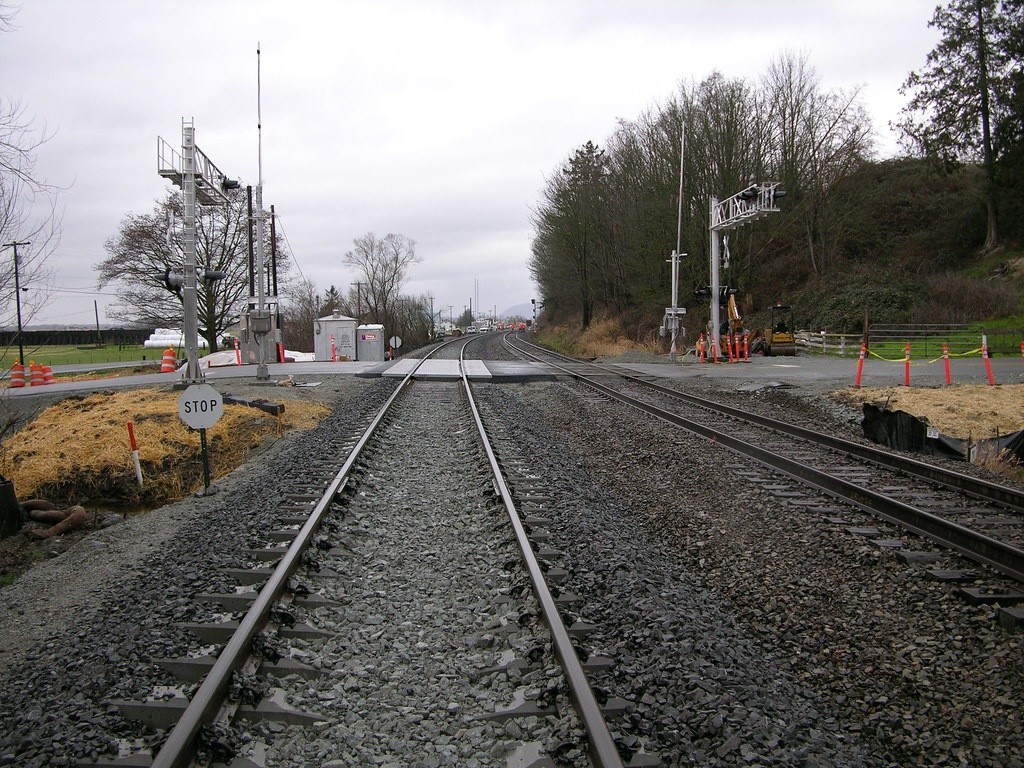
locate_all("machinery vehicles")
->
[693,284,798,356]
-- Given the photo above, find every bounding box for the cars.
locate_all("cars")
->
[466,326,489,333]
